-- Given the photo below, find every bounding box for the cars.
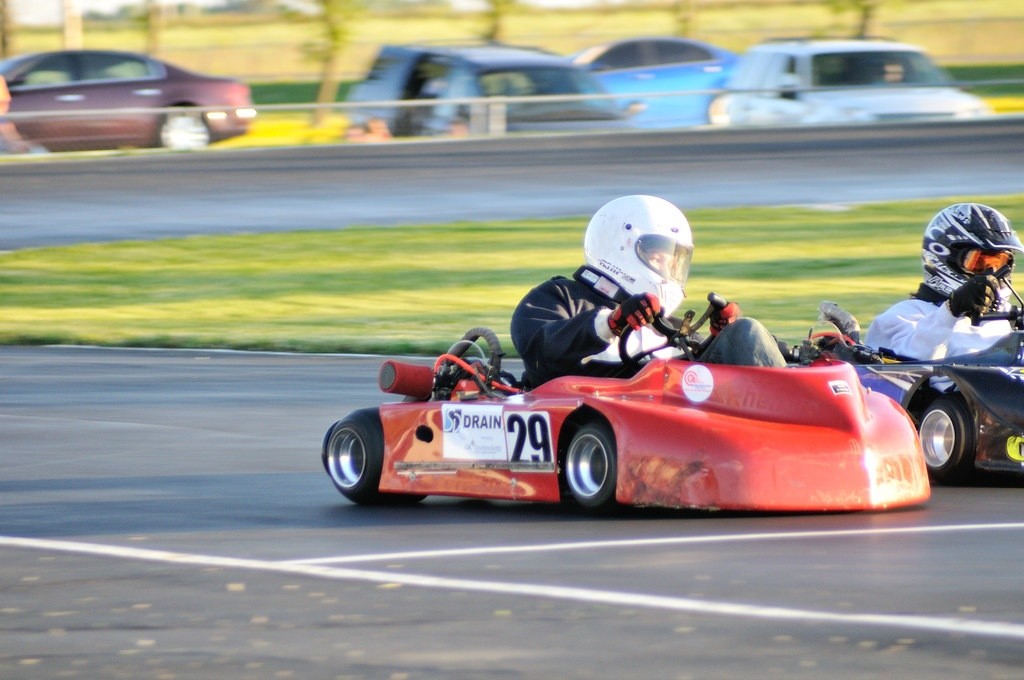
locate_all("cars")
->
[0,48,257,150]
[709,34,992,131]
[346,42,647,139]
[569,37,739,126]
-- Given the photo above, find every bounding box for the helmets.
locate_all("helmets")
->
[920,204,1023,289]
[583,194,695,318]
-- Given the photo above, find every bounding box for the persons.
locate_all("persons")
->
[864,202,1024,364]
[510,195,789,392]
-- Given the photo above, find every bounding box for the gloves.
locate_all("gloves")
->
[949,275,1000,318]
[709,302,740,336]
[607,292,661,337]
